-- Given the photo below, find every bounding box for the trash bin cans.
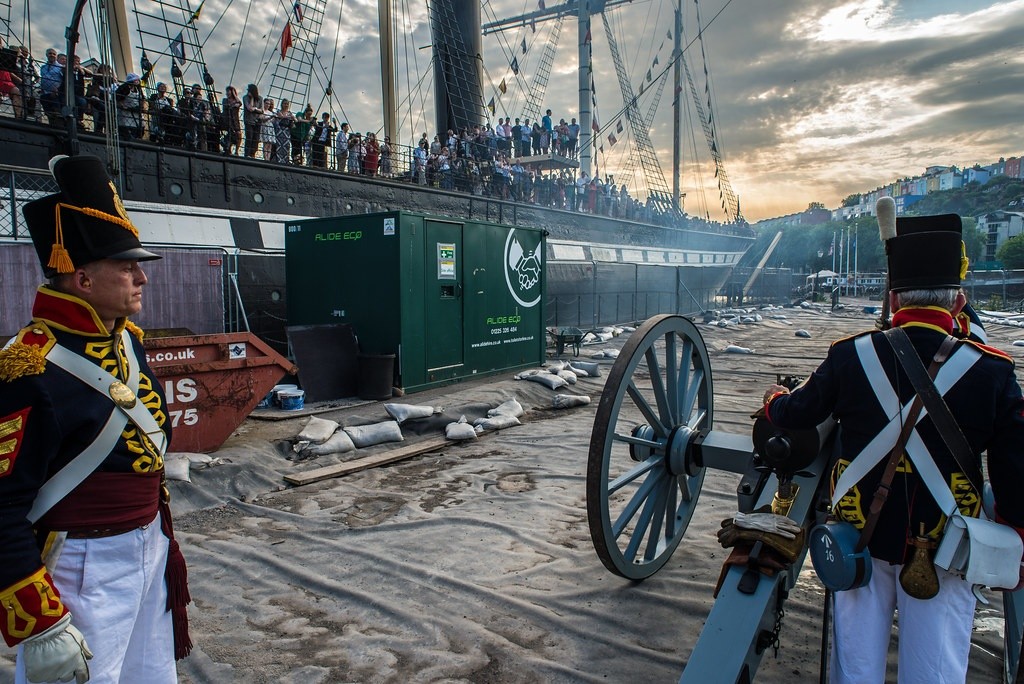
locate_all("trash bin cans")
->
[360,353,396,400]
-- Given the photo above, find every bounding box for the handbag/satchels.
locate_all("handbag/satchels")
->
[809,519,873,593]
[935,514,1022,591]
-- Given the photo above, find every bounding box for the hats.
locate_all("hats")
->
[126,73,139,82]
[876,196,968,291]
[22,155,164,279]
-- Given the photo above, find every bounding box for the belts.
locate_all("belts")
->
[65,525,147,539]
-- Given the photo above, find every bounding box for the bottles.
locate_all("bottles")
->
[898,522,940,599]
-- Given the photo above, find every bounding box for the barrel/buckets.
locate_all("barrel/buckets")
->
[277,389,305,411]
[273,384,297,406]
[254,389,273,409]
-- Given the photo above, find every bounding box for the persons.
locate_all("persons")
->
[764,214,1024,682]
[414,124,643,221]
[0,156,193,683]
[644,197,736,235]
[243,84,338,167]
[496,110,579,160]
[336,123,395,178]
[0,39,242,156]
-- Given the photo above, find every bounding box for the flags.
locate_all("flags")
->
[140,0,206,86]
[281,0,303,60]
[585,0,729,216]
[487,0,546,116]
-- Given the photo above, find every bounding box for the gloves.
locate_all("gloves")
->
[717,511,805,562]
[24,616,93,684]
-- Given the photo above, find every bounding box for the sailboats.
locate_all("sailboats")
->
[0,0,761,381]
